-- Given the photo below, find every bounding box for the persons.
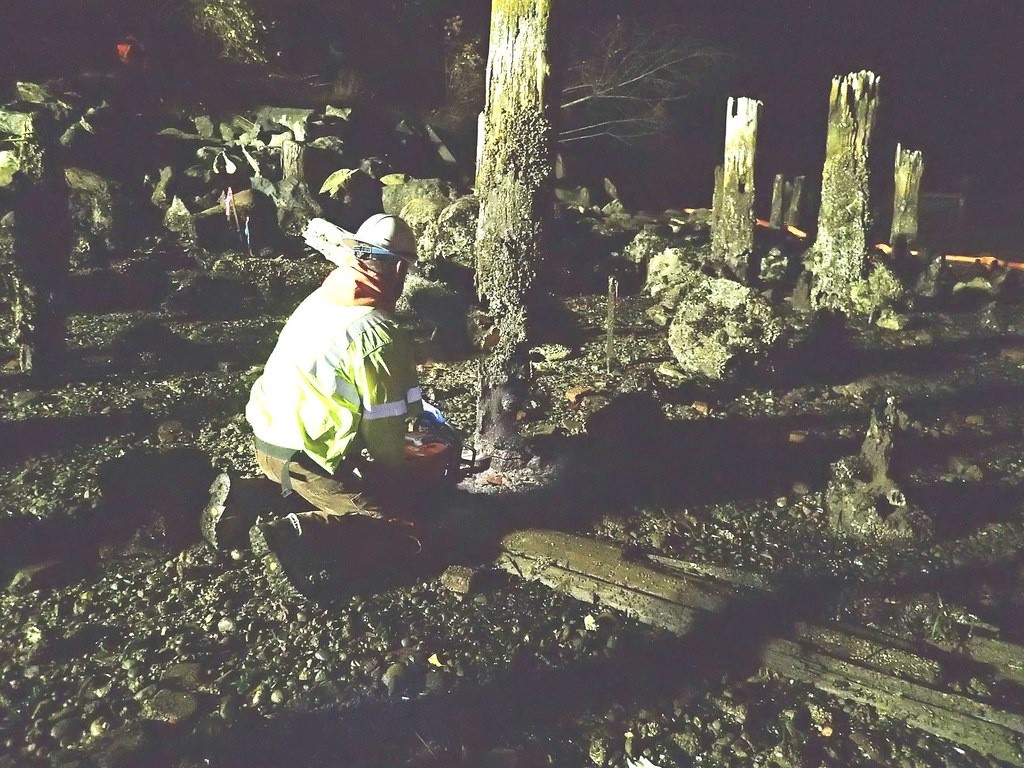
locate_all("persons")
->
[199,213,451,599]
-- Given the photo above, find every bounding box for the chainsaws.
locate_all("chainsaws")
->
[401,424,490,497]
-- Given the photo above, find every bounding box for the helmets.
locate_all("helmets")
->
[353,213,419,267]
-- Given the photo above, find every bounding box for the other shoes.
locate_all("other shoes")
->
[198,471,245,551]
[247,516,328,615]
